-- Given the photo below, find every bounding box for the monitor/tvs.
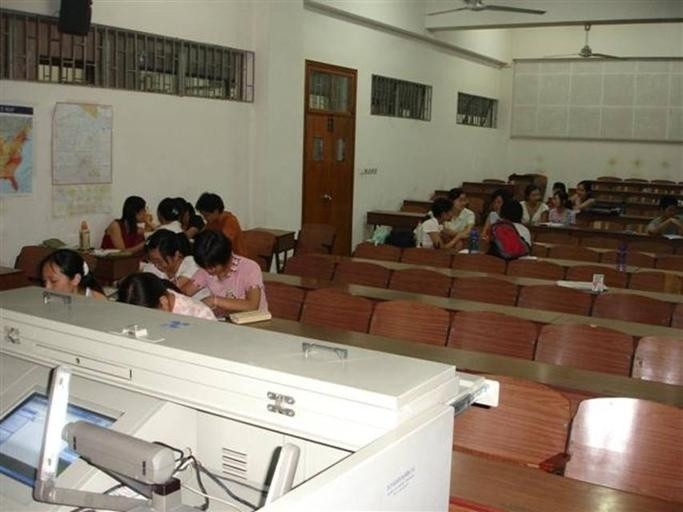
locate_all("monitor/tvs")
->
[0,385,122,498]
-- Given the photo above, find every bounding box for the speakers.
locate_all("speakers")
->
[58,0,92,36]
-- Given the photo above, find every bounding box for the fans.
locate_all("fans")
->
[424,0,548,19]
[540,23,627,62]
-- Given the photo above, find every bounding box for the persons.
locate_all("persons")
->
[647,194,683,236]
[39,192,269,322]
[412,180,597,261]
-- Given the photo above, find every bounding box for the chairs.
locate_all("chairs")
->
[258,177,682,501]
[16,246,58,283]
[241,230,277,270]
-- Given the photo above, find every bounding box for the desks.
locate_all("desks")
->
[451,449,676,511]
[1,285,459,453]
[71,247,139,291]
[2,266,24,291]
[255,227,294,271]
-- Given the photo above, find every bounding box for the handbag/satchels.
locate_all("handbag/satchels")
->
[385,226,413,248]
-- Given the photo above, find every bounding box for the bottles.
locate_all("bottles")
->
[616,244,627,272]
[470,228,480,250]
[564,211,572,228]
[78,220,90,250]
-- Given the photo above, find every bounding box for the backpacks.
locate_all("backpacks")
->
[489,220,531,259]
